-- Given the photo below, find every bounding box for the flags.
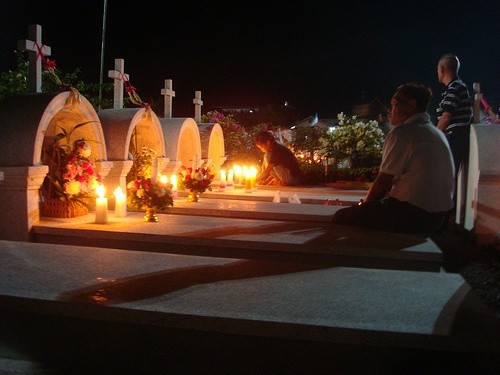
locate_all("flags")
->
[308,113,320,126]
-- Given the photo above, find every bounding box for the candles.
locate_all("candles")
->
[221,165,257,189]
[115,194,126,216]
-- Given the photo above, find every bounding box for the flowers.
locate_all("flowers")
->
[179,165,215,191]
[44,119,100,198]
[128,178,173,207]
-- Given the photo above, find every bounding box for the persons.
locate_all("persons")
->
[436,53,474,172]
[334,82,457,241]
[252,131,304,186]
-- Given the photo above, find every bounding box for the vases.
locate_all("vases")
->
[144,207,159,222]
[187,190,201,201]
[45,197,88,217]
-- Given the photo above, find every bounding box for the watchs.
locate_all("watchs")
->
[357,199,365,207]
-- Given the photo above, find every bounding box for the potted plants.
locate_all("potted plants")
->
[95,184,108,223]
